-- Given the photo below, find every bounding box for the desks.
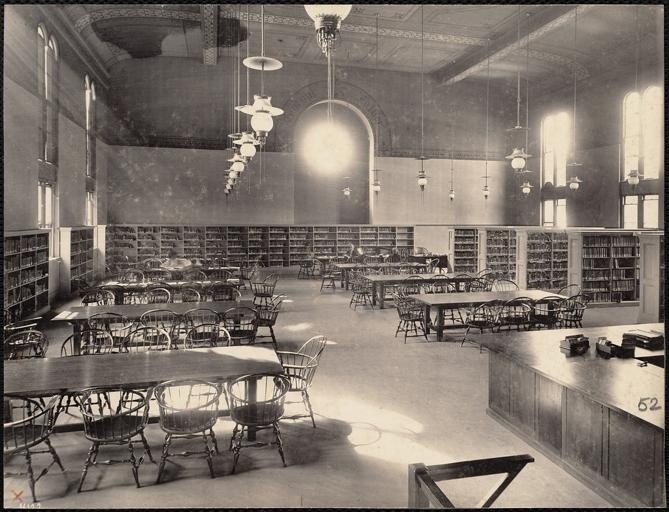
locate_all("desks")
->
[460,322,667,507]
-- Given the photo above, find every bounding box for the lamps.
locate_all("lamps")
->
[220,4,644,201]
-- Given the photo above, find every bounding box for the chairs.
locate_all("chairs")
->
[330,240,593,349]
[3,246,331,505]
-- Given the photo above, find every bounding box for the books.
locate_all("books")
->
[487,229,516,280]
[659,238,667,321]
[270,228,313,266]
[454,229,478,274]
[71,230,94,293]
[229,228,268,269]
[106,227,136,276]
[314,227,359,264]
[527,231,568,290]
[361,227,396,262]
[138,228,182,270]
[583,235,640,303]
[184,227,226,266]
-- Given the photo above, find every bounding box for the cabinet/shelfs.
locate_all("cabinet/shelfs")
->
[4,227,54,322]
[58,217,418,299]
[446,223,665,325]
